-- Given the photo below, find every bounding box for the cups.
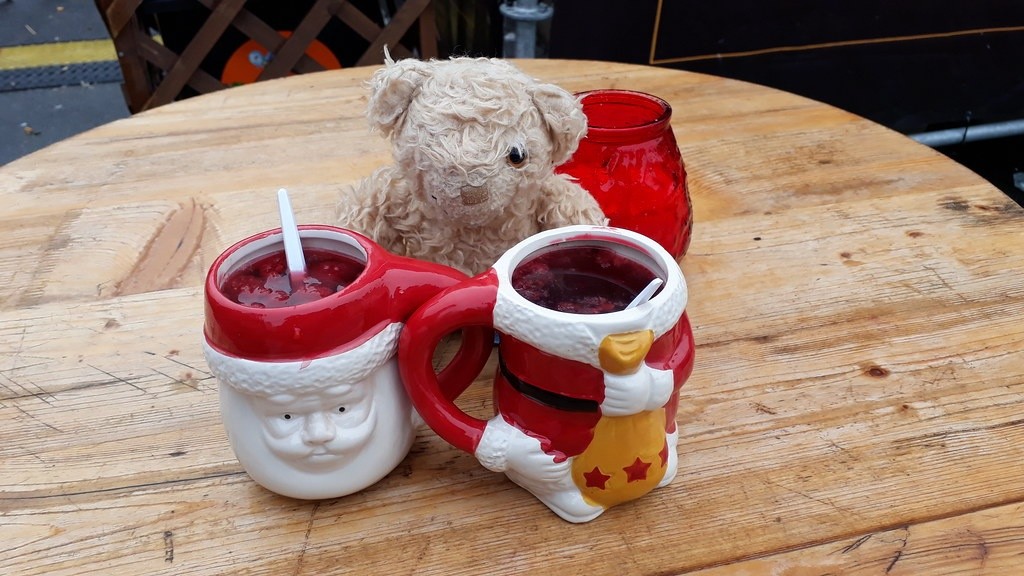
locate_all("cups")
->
[400,224,698,523]
[198,224,493,501]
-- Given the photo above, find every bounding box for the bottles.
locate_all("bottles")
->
[554,89,693,267]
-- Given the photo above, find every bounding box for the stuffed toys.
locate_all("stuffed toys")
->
[328,58,607,277]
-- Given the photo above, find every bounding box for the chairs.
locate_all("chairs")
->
[92,0,432,116]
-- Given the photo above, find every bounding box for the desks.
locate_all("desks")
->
[0,56,1024,576]
